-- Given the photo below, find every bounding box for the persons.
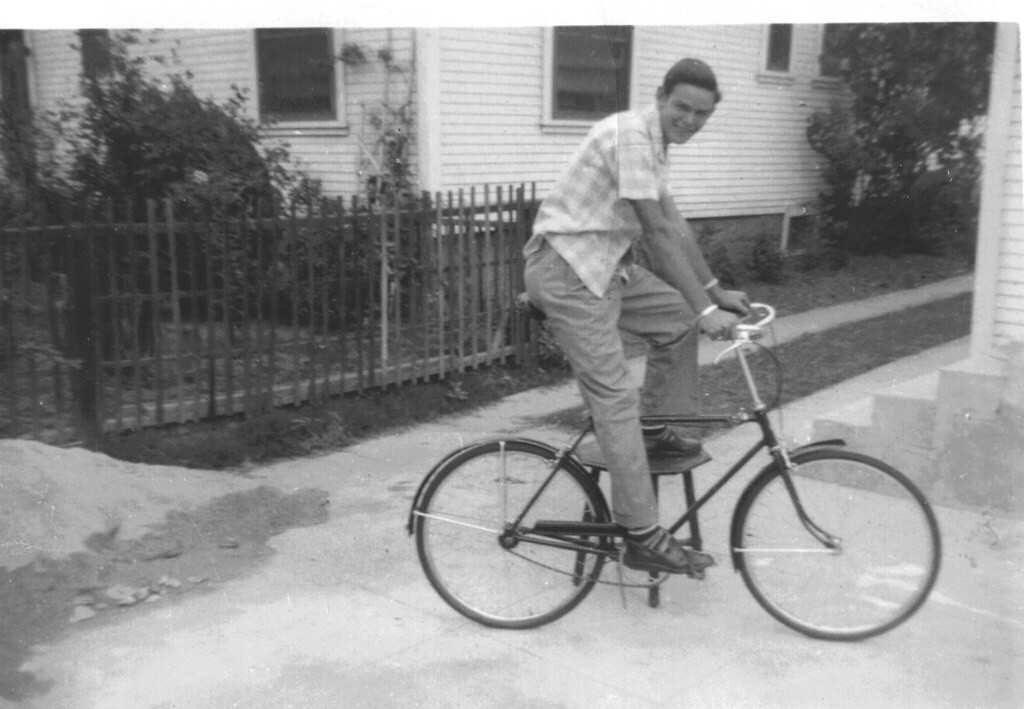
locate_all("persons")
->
[523,57,752,574]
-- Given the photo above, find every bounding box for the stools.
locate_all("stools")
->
[571,435,713,609]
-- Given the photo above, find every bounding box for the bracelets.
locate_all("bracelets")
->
[694,303,720,322]
[702,277,721,291]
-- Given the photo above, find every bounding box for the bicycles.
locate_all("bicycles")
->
[405,303,944,643]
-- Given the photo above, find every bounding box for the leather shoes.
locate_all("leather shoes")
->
[622,526,714,574]
[641,423,702,455]
